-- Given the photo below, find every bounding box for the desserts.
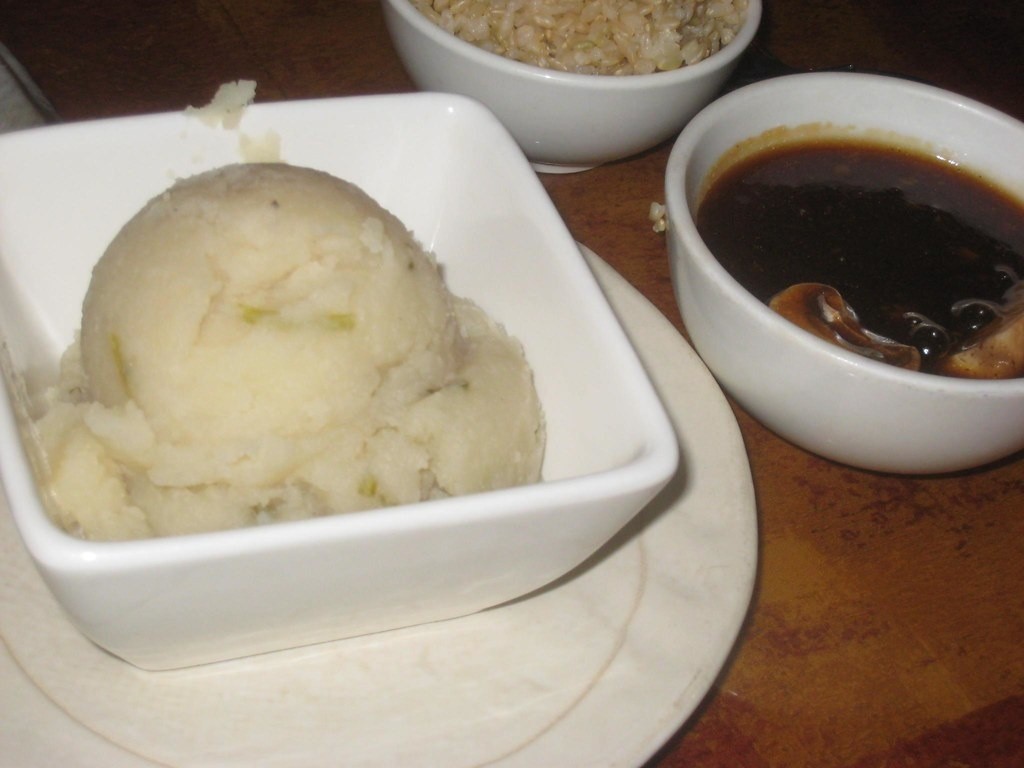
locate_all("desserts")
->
[17,73,548,545]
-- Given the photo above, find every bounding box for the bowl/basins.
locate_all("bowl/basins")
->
[383,0,763,173]
[666,72,1024,475]
[0,92,681,670]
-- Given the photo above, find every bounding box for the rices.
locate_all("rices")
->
[409,0,753,76]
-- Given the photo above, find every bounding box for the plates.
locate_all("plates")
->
[0,241,759,768]
